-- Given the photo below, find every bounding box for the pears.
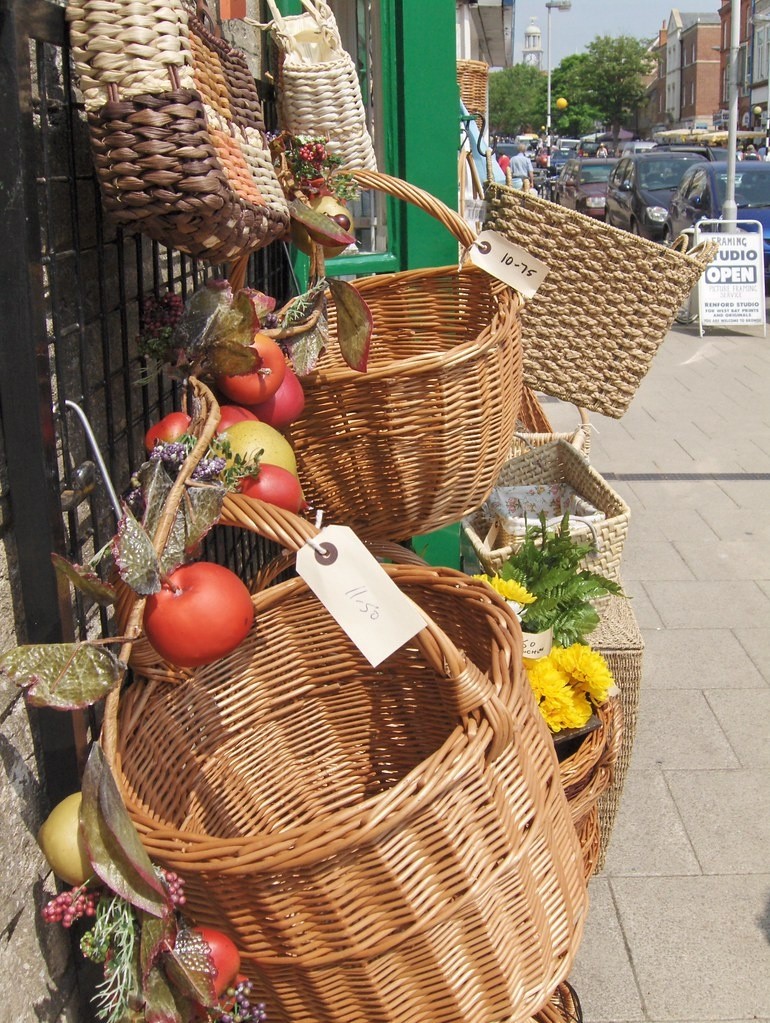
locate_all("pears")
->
[38,790,114,889]
[214,422,296,488]
[289,194,355,258]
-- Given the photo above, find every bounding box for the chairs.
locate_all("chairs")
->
[642,162,663,183]
[665,163,682,184]
[580,171,593,181]
[741,173,770,202]
[717,177,728,196]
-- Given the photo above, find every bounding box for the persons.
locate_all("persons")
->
[596,142,607,158]
[535,147,548,168]
[544,141,551,155]
[575,143,584,157]
[510,144,534,187]
[703,140,768,160]
[497,152,510,175]
[522,124,545,148]
[546,131,574,147]
[492,135,514,149]
[645,161,672,179]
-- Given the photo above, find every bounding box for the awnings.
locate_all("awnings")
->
[609,129,633,138]
[580,133,605,142]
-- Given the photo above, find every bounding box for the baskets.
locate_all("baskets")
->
[460,147,719,465]
[264,169,525,544]
[458,437,630,589]
[243,0,378,193]
[456,60,489,129]
[98,493,588,1023]
[244,542,643,886]
[65,0,290,265]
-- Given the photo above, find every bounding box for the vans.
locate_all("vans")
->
[621,141,658,158]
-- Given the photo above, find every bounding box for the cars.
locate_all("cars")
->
[513,134,623,204]
[662,160,770,294]
[492,143,526,160]
[736,152,762,161]
[604,153,711,244]
[554,158,623,221]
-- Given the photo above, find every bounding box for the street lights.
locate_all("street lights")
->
[545,0,571,137]
[754,106,762,127]
[556,97,568,137]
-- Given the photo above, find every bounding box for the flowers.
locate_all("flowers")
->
[471,505,629,734]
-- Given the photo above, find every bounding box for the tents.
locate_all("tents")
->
[655,128,766,141]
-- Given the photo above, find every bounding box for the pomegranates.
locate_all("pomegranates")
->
[169,926,248,1005]
[234,463,313,516]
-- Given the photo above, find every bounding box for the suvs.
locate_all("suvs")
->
[649,143,740,164]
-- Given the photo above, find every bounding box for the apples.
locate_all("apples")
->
[145,335,304,453]
[144,562,255,669]
